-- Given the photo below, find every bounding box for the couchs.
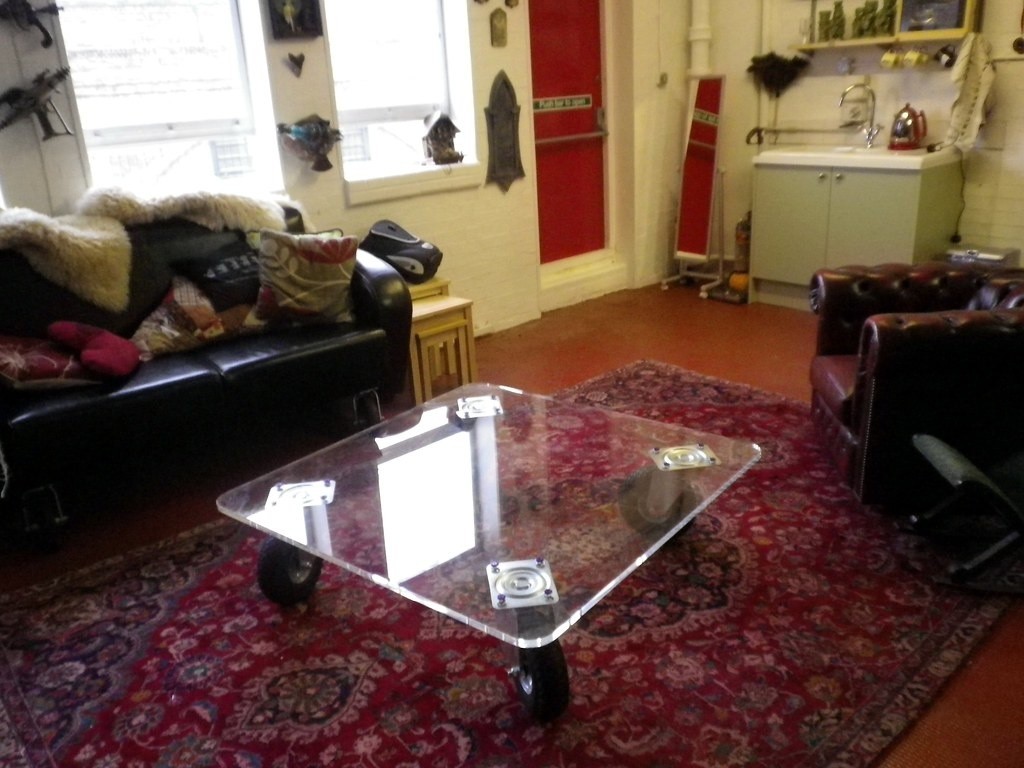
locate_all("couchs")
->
[807,264,1024,530]
[0,205,416,553]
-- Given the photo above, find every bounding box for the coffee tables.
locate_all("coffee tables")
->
[213,387,764,720]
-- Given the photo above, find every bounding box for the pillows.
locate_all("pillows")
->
[238,224,357,326]
[44,320,140,384]
[0,336,103,389]
[168,256,261,313]
[131,275,225,361]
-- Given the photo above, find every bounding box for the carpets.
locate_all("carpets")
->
[0,358,1024,768]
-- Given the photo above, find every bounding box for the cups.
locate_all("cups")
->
[880,44,957,67]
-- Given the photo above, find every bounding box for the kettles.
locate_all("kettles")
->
[887,103,929,149]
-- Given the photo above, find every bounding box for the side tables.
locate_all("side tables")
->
[402,277,478,407]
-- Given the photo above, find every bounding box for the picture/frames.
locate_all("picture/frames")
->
[267,0,324,39]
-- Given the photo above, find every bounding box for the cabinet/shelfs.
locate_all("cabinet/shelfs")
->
[788,0,983,52]
[747,163,963,313]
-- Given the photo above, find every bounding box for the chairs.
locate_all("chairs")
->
[909,432,1024,585]
